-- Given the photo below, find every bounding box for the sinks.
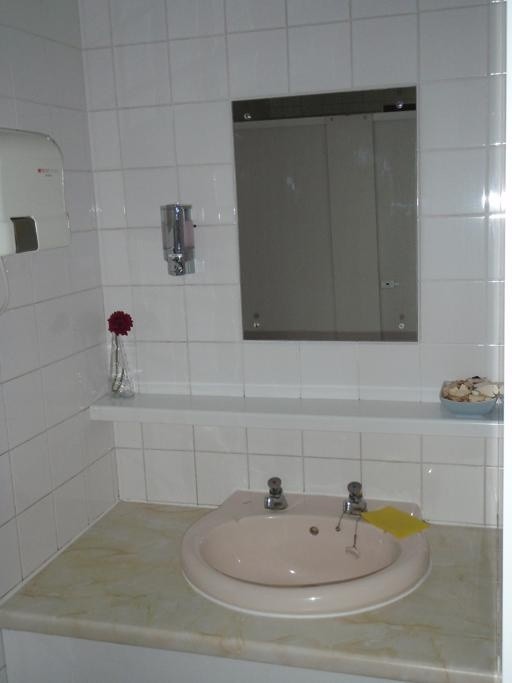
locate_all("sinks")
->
[179,489,432,618]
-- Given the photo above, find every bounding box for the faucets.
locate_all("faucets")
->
[343,482,367,516]
[262,477,288,509]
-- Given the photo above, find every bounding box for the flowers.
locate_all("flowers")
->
[107,310,133,391]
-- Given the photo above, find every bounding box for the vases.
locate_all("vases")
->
[107,336,134,399]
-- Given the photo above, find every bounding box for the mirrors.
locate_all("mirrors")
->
[230,87,416,343]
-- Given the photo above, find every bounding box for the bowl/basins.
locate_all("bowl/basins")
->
[439,396,498,419]
